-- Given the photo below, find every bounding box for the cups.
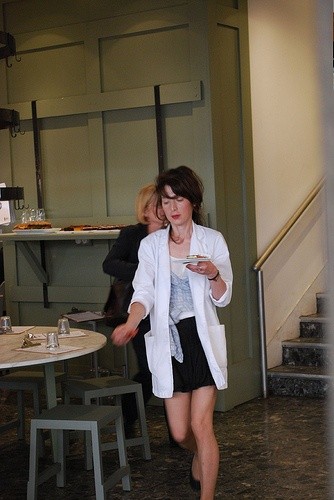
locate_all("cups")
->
[58,318,70,333]
[47,332,59,348]
[0,316,12,333]
[22,208,45,224]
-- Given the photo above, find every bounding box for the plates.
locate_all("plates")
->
[177,258,210,264]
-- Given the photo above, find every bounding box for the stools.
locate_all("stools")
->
[62,318,130,404]
[0,370,151,500]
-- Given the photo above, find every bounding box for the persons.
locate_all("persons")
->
[112,166,233,500]
[101,185,168,439]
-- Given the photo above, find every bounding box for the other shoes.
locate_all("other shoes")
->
[123,418,137,437]
[188,453,201,490]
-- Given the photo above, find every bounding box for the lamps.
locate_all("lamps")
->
[0,25,29,210]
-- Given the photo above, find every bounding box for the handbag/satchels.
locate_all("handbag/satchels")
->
[103,280,150,326]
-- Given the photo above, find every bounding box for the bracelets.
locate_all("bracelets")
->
[208,269,219,281]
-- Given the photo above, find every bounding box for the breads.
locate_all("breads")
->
[60,224,125,231]
[14,221,51,229]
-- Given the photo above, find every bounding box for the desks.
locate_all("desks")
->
[0,324,107,471]
[0,231,122,283]
